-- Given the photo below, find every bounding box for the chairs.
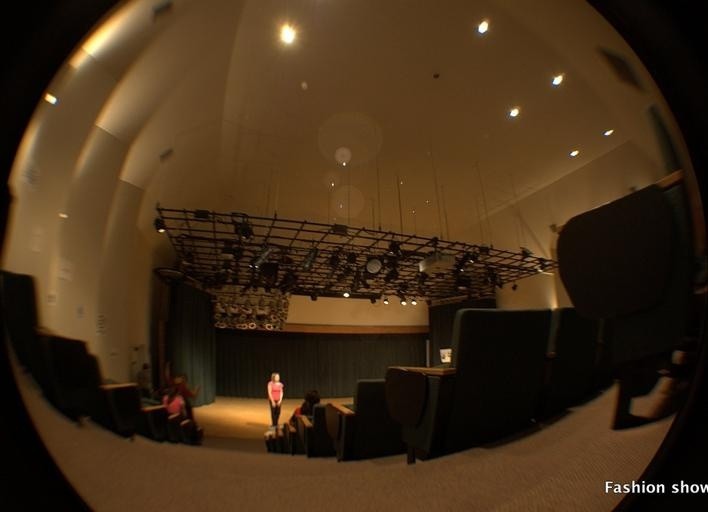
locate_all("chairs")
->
[263,163,704,465]
[1,270,204,444]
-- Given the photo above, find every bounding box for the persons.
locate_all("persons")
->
[288,389,320,427]
[136,363,202,423]
[267,373,284,426]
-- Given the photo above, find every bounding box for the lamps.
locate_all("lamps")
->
[153,217,165,233]
